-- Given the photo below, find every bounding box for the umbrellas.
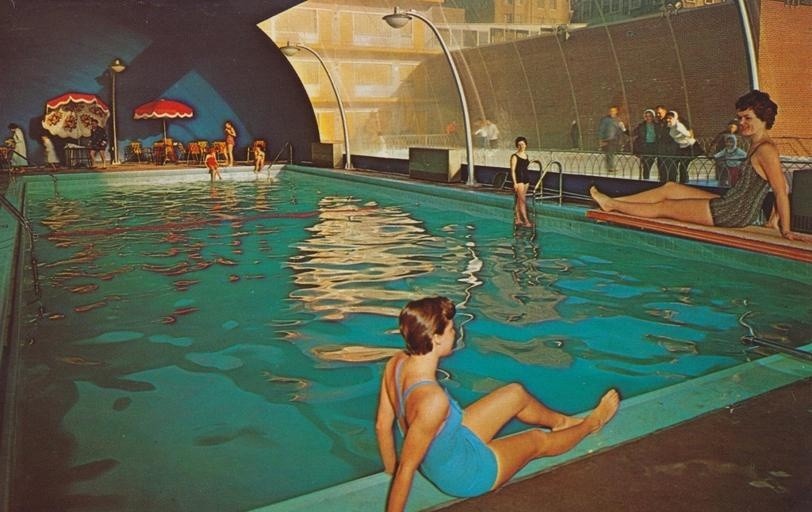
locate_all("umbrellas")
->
[41,93,111,144]
[134,99,193,161]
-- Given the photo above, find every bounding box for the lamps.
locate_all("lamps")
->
[660,2,682,19]
[557,24,570,40]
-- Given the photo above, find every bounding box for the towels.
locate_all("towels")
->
[64,143,85,148]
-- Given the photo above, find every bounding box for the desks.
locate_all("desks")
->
[65,148,93,169]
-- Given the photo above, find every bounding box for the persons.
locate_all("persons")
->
[254,144,266,173]
[205,145,222,182]
[589,90,812,242]
[570,119,579,147]
[375,296,620,512]
[41,133,60,170]
[223,120,237,167]
[88,120,108,169]
[511,137,534,229]
[473,118,499,147]
[8,123,28,173]
[446,120,458,134]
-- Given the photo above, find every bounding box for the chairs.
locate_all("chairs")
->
[131,138,228,167]
[247,140,266,161]
